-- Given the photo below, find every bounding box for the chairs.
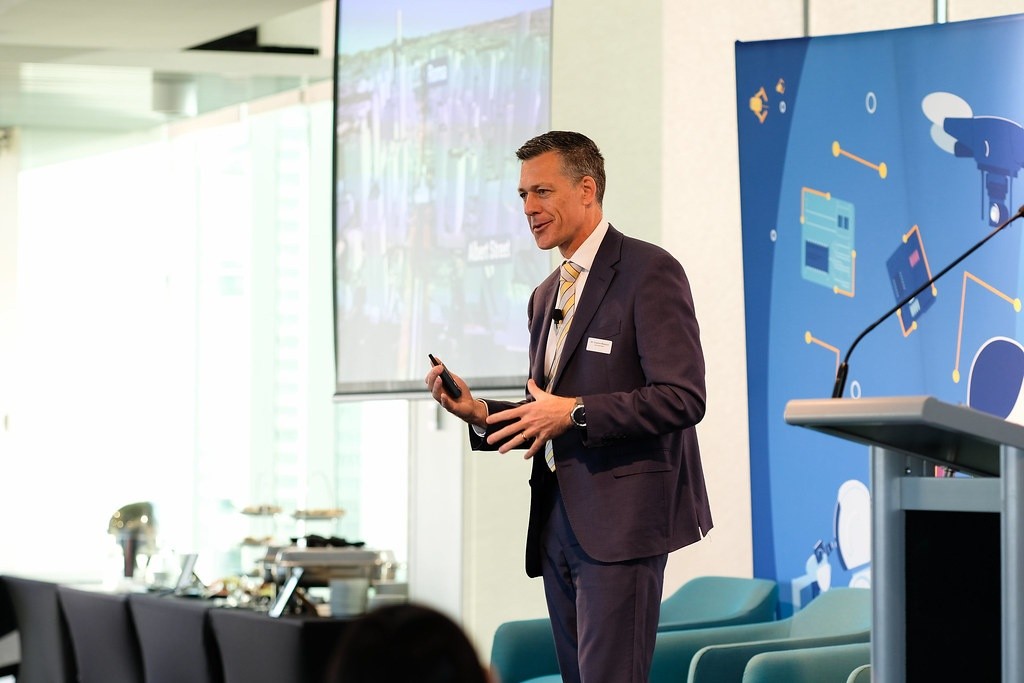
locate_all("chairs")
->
[0,575,871,683]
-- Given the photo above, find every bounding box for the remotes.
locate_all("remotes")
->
[428,354,462,398]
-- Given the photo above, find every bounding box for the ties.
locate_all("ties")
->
[543,261,583,472]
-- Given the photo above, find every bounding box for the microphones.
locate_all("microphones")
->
[552,309,564,325]
[832,203,1024,399]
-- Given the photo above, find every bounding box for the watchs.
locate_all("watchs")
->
[570,396,587,430]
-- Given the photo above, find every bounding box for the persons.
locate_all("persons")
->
[425,131,713,683]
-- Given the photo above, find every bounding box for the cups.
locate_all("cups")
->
[329,578,369,616]
[375,583,408,596]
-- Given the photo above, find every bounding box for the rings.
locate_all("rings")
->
[520,431,529,442]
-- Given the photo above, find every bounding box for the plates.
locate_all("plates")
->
[368,595,404,613]
